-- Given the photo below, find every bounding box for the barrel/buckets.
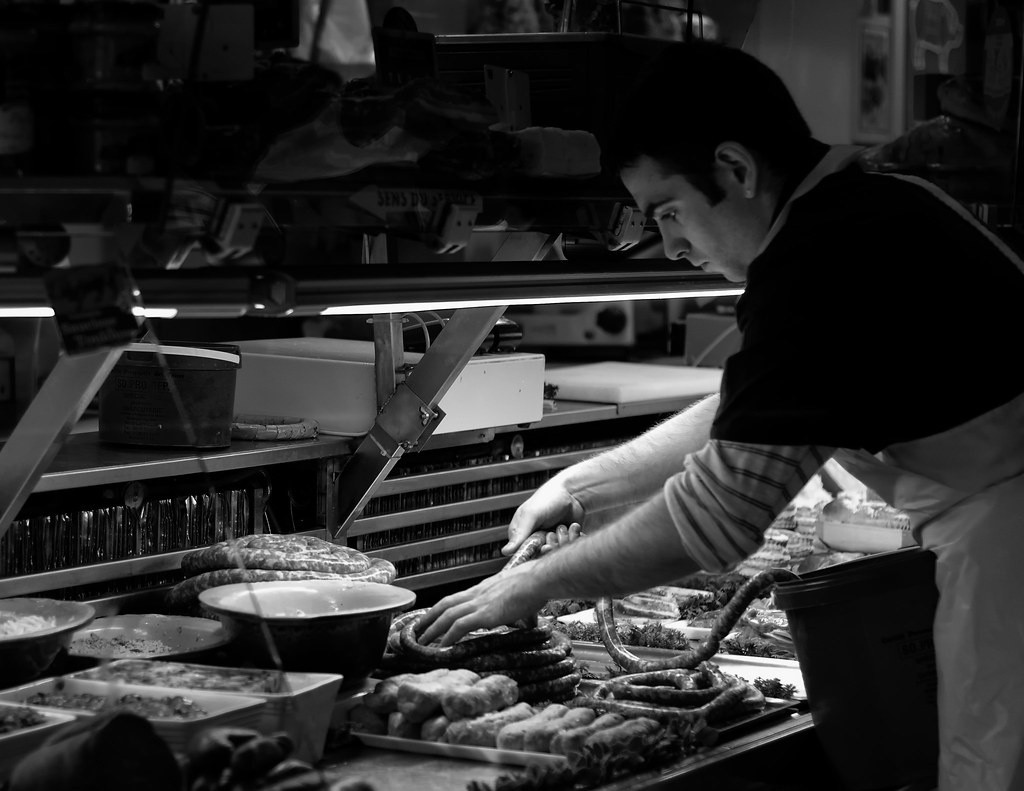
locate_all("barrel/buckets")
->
[772,548,938,791]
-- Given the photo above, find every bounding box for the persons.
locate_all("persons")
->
[412,39,1024,791]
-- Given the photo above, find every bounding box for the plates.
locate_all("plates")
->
[69,614,227,661]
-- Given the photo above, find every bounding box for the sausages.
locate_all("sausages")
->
[163,533,814,763]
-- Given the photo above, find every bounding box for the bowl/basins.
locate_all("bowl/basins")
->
[0,596,98,690]
[197,578,416,700]
